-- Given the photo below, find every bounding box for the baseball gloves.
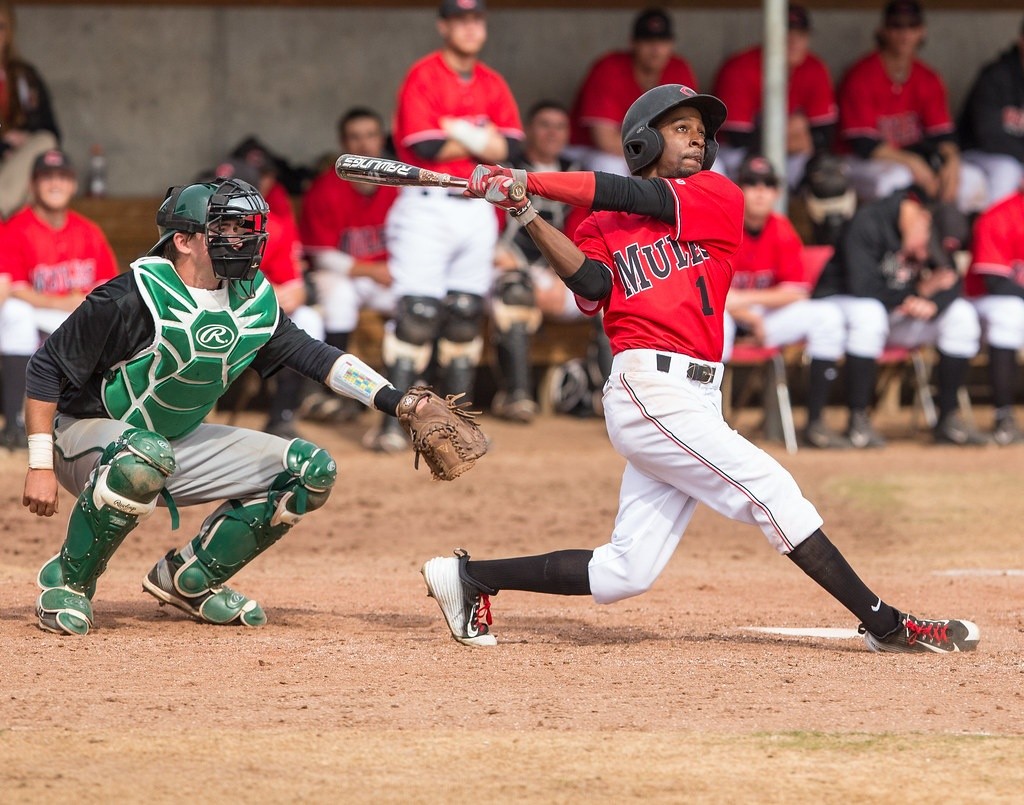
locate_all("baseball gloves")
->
[397,386,488,482]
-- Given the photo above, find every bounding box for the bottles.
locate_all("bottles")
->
[86,144,110,198]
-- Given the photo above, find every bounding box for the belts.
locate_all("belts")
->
[657,354,715,384]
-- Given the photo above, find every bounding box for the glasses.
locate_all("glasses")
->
[741,176,776,188]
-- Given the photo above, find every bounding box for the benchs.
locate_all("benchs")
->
[68,197,1024,367]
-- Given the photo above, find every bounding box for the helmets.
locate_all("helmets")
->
[145,176,270,300]
[621,84,727,176]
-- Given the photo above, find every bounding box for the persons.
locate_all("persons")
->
[956,16,1024,208]
[568,8,725,178]
[0,1,60,215]
[490,102,612,420]
[194,137,337,439]
[814,186,986,447]
[421,83,980,653]
[966,176,1023,445]
[711,4,838,205]
[837,0,960,207]
[378,0,526,455]
[25,179,487,634]
[721,157,893,448]
[0,153,122,447]
[301,108,400,427]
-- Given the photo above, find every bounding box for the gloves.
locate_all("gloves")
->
[463,162,528,198]
[484,175,532,217]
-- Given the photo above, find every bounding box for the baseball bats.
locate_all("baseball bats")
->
[335,153,526,202]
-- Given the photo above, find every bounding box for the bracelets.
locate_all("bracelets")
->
[374,385,404,417]
[27,433,53,469]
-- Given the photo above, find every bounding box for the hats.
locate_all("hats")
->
[633,9,676,41]
[33,150,74,175]
[439,0,486,16]
[212,160,261,193]
[788,7,809,28]
[884,2,923,28]
[738,155,778,181]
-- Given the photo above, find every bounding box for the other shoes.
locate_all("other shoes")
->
[486,392,540,423]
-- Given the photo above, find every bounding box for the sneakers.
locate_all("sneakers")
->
[421,548,499,647]
[142,547,264,626]
[857,609,980,653]
[805,411,892,448]
[34,590,75,635]
[931,408,1019,447]
